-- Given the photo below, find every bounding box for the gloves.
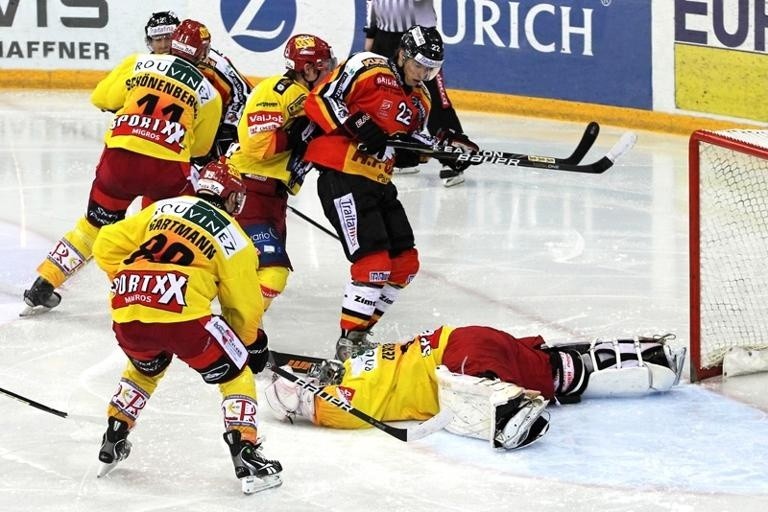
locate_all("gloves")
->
[393,149,421,169]
[216,124,237,153]
[435,126,479,153]
[344,111,387,159]
[287,116,323,147]
[245,328,269,374]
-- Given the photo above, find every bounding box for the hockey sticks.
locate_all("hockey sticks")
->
[470,122,600,165]
[385,131,640,175]
[267,361,457,444]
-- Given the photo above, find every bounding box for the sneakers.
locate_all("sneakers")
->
[223,430,283,479]
[23,277,62,308]
[439,163,458,178]
[98,416,131,464]
[335,330,363,365]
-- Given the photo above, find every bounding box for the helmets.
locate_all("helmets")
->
[194,161,247,220]
[145,11,445,74]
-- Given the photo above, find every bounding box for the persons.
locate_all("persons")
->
[265,328,688,450]
[217,36,334,318]
[141,12,256,166]
[363,0,479,180]
[306,23,476,360]
[88,160,287,494]
[22,20,222,308]
